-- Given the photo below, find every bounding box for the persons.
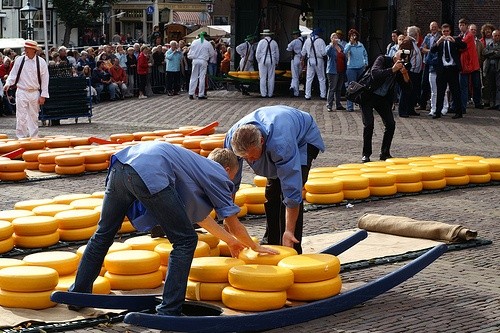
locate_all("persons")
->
[224,105,325,254]
[359,50,413,163]
[67,140,280,317]
[0,18,500,138]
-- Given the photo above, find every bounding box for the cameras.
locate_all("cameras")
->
[398,50,413,70]
[444,37,451,40]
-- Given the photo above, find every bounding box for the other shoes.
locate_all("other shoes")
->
[336,106,346,110]
[168,91,174,96]
[327,108,333,112]
[393,100,499,118]
[199,95,208,100]
[289,88,294,97]
[380,155,393,160]
[361,156,370,163]
[189,95,194,99]
[174,91,180,95]
[139,94,147,99]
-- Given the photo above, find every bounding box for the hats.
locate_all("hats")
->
[290,29,302,35]
[311,27,324,35]
[260,29,275,35]
[336,30,344,35]
[23,39,41,51]
[244,34,258,41]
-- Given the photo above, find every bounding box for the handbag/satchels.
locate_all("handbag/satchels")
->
[346,81,372,104]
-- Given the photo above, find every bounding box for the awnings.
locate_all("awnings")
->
[172,11,211,25]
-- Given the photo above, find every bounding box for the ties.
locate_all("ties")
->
[444,40,451,63]
